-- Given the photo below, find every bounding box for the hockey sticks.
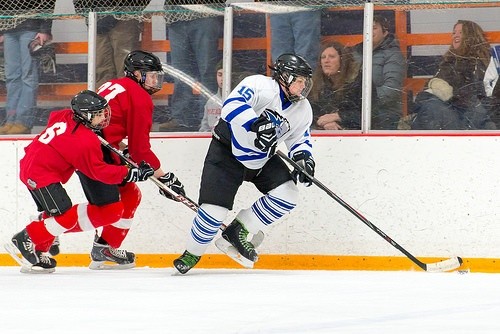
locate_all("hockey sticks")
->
[96,133,269,249]
[273,149,464,272]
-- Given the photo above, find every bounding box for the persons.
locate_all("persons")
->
[0,0,57,133]
[73,0,150,91]
[40,51,185,264]
[159,0,224,131]
[199,56,241,132]
[268,0,321,103]
[409,20,499,129]
[173,53,315,274]
[11,90,155,269]
[312,42,379,130]
[352,15,404,130]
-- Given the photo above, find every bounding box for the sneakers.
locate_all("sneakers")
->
[90,229,136,270]
[172,251,201,274]
[214,219,259,269]
[20,255,57,274]
[4,228,39,268]
[30,212,60,256]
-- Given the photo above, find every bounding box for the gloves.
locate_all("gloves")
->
[251,118,278,159]
[289,151,316,187]
[126,160,154,183]
[156,171,186,203]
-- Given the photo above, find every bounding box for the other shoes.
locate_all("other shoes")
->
[0,121,32,134]
[159,119,184,132]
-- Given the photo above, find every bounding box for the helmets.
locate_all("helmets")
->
[124,50,163,85]
[271,53,313,82]
[71,89,109,123]
